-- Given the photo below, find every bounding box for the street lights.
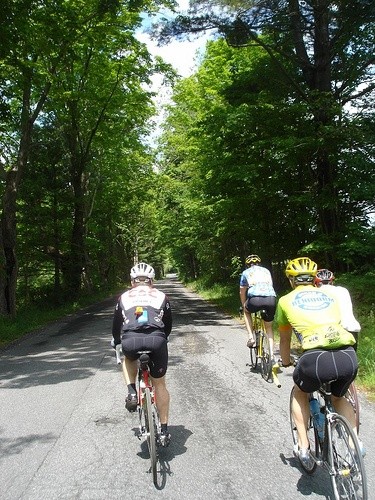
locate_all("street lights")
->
[134,237,151,265]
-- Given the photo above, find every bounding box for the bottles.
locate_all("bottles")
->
[309,398,321,429]
[318,406,326,438]
[140,376,152,409]
[256,333,260,347]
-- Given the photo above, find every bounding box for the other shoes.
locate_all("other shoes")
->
[271,355,275,365]
[247,338,256,347]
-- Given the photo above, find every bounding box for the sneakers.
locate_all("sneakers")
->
[159,431,172,447]
[355,442,367,465]
[292,442,311,462]
[125,393,139,412]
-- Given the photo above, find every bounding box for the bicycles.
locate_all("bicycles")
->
[115,340,172,484]
[278,358,368,500]
[239,306,273,381]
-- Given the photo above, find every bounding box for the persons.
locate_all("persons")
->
[278,258,366,460]
[111,263,173,447]
[240,255,276,362]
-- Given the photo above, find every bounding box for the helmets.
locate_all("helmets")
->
[244,254,261,266]
[285,257,318,283]
[316,268,335,283]
[130,262,155,284]
